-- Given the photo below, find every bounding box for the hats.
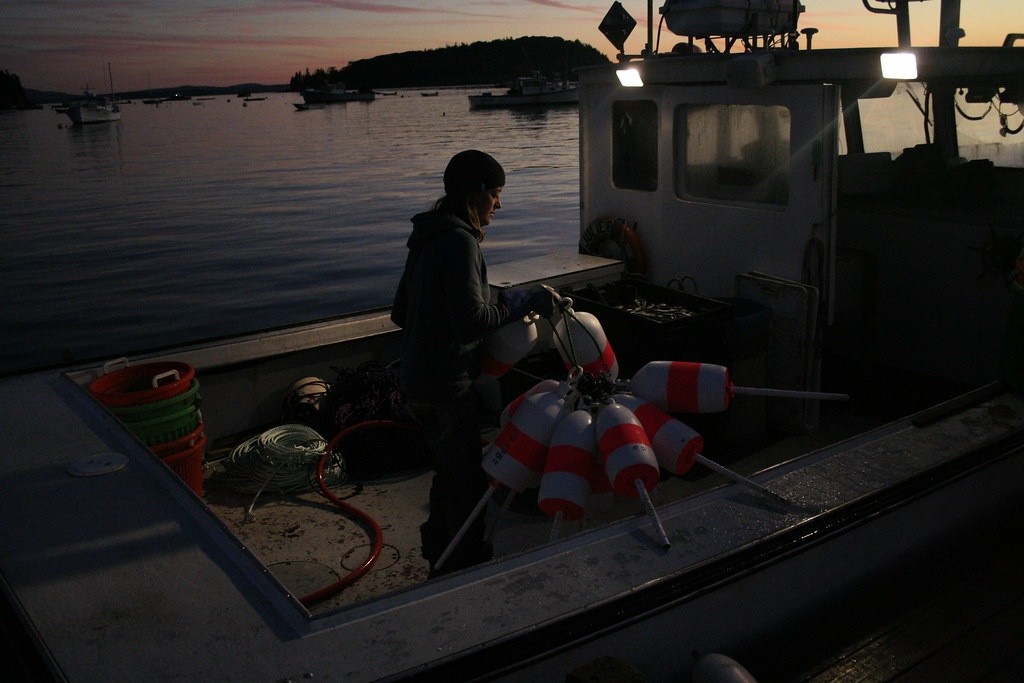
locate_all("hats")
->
[444,150,505,200]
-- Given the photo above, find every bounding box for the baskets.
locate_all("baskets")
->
[90,357,205,499]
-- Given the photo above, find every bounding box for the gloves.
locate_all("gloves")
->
[497,286,553,322]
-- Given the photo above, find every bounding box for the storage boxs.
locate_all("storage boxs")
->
[560,278,733,480]
[499,348,571,411]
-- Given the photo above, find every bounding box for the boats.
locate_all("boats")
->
[0,1,1024,683]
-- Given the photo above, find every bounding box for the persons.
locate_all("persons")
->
[392,150,553,561]
